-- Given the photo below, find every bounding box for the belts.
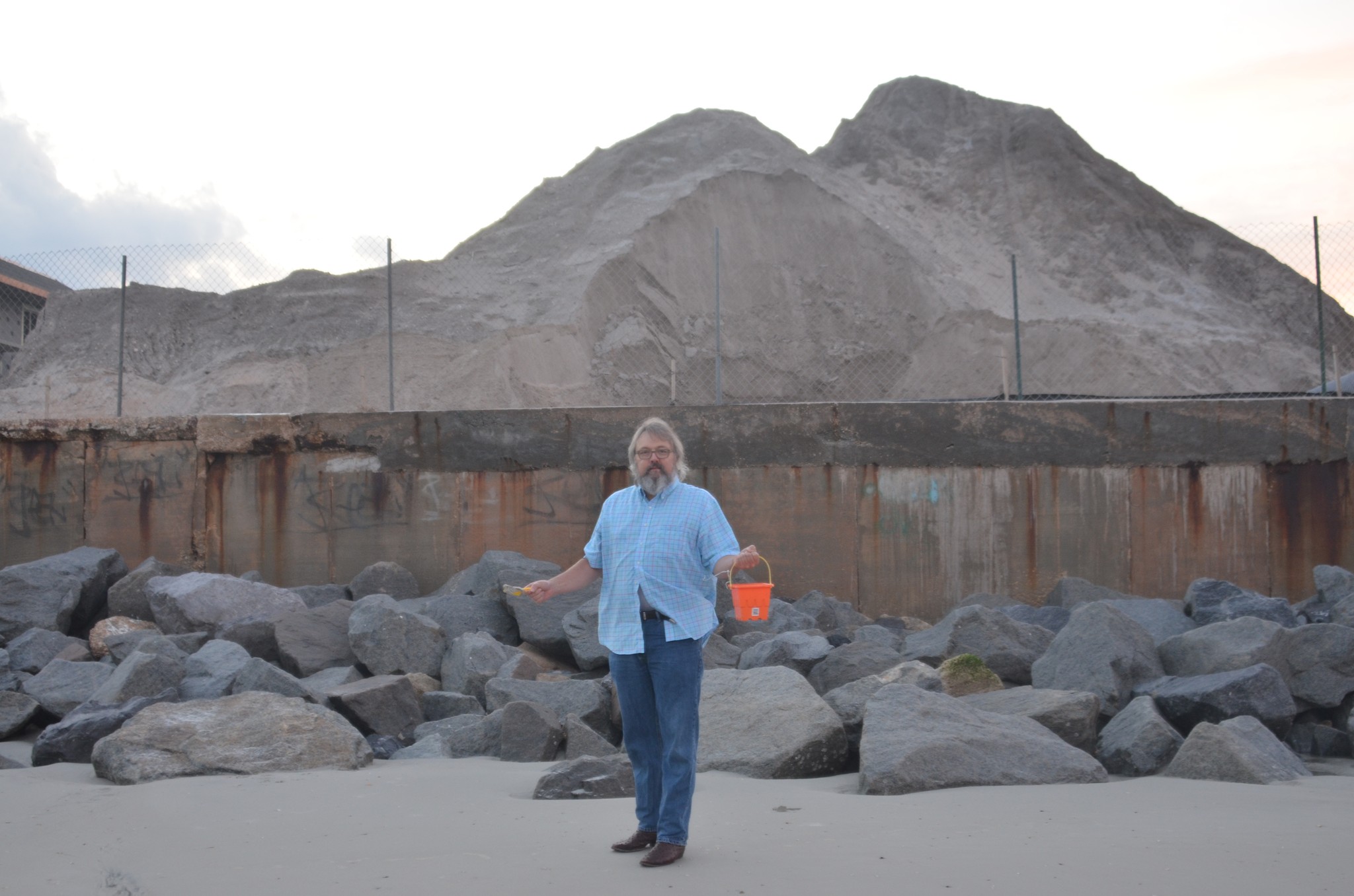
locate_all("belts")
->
[640,611,676,624]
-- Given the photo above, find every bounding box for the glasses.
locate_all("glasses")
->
[635,449,676,459]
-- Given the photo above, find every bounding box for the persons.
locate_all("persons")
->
[522,420,758,866]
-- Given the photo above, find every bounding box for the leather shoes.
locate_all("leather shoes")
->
[611,829,657,852]
[639,842,685,867]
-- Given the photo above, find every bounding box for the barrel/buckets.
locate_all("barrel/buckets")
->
[728,555,774,622]
